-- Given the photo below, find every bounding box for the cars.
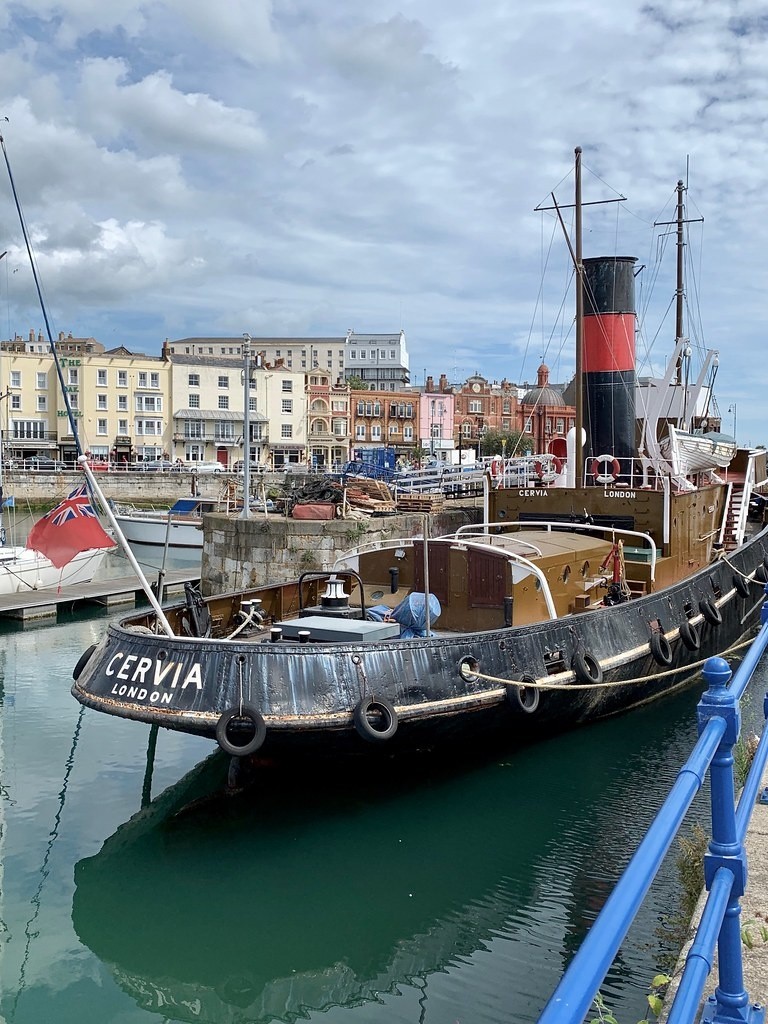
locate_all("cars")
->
[19,456,69,471]
[187,462,227,474]
[233,459,300,474]
[425,460,457,476]
[141,459,175,471]
[76,460,111,471]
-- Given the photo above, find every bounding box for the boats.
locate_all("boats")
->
[0,482,116,594]
[109,479,293,548]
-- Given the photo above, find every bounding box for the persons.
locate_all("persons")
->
[119,455,130,474]
[107,456,118,473]
[175,456,184,472]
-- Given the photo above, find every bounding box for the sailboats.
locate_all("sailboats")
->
[72,144,768,758]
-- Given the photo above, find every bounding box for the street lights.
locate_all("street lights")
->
[727,403,737,439]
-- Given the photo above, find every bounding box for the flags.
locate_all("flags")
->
[25,472,119,569]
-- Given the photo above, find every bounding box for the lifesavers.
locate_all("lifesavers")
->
[651,633,673,665]
[734,574,750,598]
[72,644,98,680]
[505,672,540,714]
[764,554,768,569]
[354,695,398,744]
[757,566,768,588]
[700,598,722,625]
[574,650,603,684]
[535,454,561,482]
[680,622,700,651]
[492,454,504,482]
[592,454,621,484]
[216,705,268,758]
